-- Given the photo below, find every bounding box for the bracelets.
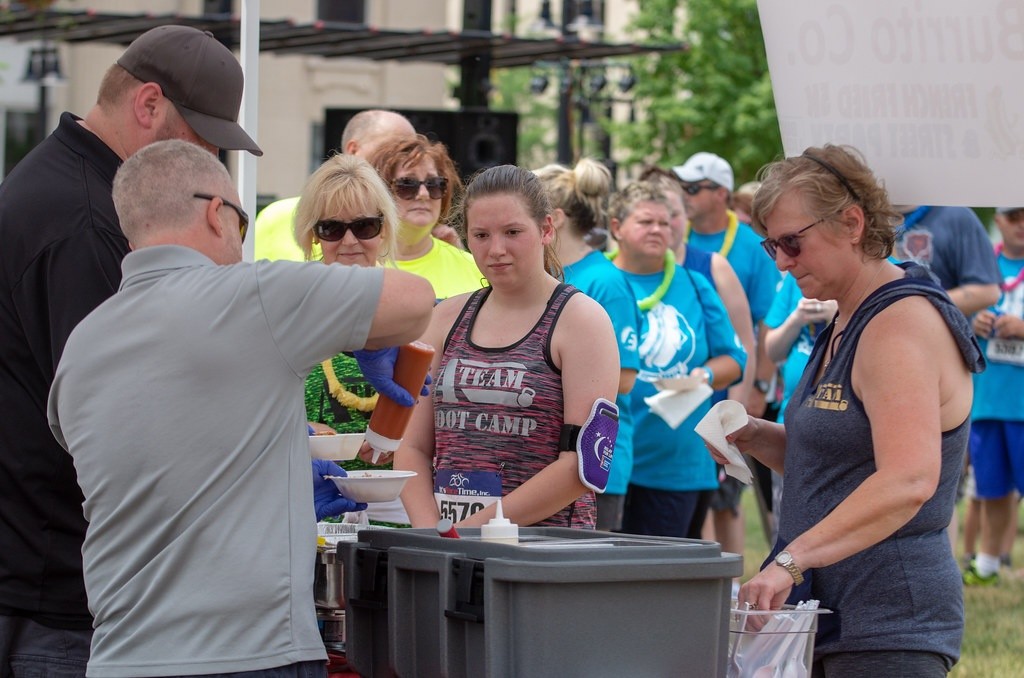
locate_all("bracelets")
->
[700,363,715,388]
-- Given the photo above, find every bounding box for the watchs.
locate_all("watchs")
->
[775,550,806,586]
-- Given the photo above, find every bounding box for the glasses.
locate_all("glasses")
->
[681,183,719,194]
[760,219,826,261]
[391,176,448,200]
[194,192,249,244]
[314,208,384,242]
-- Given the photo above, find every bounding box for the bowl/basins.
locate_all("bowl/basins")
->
[323,470,418,503]
[309,431,366,461]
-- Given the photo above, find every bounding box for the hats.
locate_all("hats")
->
[118,25,263,157]
[671,152,733,192]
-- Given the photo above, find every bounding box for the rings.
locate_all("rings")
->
[743,602,756,610]
[816,305,823,311]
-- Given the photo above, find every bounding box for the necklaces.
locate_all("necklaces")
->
[822,315,840,369]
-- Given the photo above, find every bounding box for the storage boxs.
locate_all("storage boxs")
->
[336,525,745,678]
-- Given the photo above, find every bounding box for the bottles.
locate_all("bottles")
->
[367,338,436,464]
[480,500,520,545]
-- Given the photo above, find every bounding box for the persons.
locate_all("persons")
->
[255,109,493,300]
[293,154,401,436]
[704,142,984,678]
[46,140,434,678]
[0,24,368,678]
[393,163,621,533]
[494,152,1023,620]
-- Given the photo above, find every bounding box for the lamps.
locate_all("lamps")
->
[530,55,638,94]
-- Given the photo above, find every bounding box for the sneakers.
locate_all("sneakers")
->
[961,554,1000,586]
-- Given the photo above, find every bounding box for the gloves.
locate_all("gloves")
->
[354,345,432,407]
[311,458,368,522]
